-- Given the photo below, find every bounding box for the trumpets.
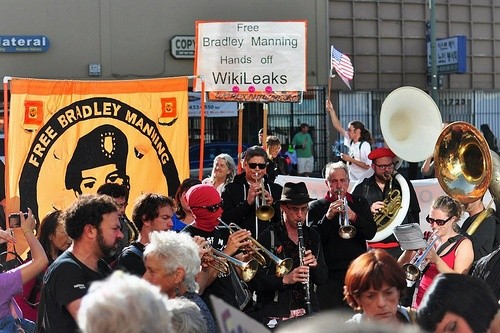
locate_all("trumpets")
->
[217,216,293,277]
[192,237,259,283]
[337,191,356,239]
[401,226,442,288]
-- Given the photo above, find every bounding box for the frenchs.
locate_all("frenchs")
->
[366,86,443,243]
[434,121,500,235]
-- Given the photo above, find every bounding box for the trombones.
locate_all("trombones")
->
[255,172,274,241]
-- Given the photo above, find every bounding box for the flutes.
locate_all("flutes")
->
[297,219,315,319]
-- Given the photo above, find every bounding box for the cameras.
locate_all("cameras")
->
[9,213,28,228]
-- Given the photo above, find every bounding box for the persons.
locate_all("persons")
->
[76,268,176,333]
[417,271,500,333]
[325,101,375,196]
[479,124,498,154]
[140,229,222,333]
[344,247,431,333]
[0,134,500,333]
[292,123,314,178]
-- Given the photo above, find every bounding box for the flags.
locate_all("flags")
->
[330,45,354,80]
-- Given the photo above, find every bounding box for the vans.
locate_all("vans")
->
[189,141,249,176]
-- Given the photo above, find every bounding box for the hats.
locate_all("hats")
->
[273,181,318,205]
[367,148,395,160]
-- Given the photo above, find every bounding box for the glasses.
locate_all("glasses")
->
[425,214,452,226]
[191,198,225,213]
[283,201,310,212]
[375,163,394,170]
[249,163,266,170]
